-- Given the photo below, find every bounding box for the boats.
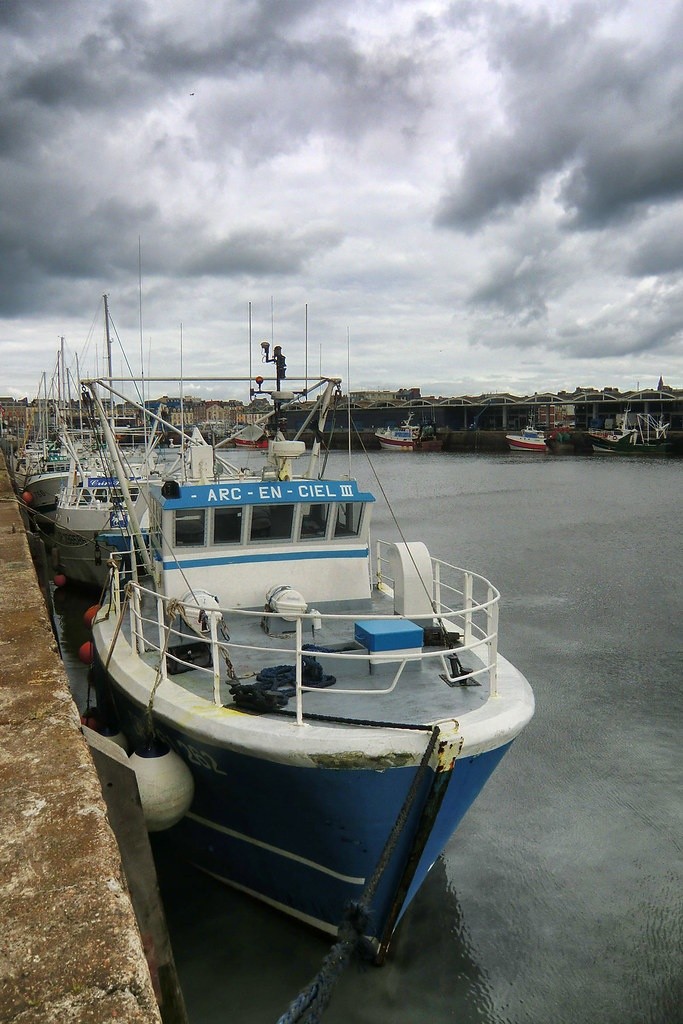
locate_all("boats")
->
[232,434,270,449]
[505,426,552,451]
[581,409,678,456]
[372,426,416,451]
[75,300,537,963]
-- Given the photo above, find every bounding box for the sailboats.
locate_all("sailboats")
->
[0,295,230,589]
